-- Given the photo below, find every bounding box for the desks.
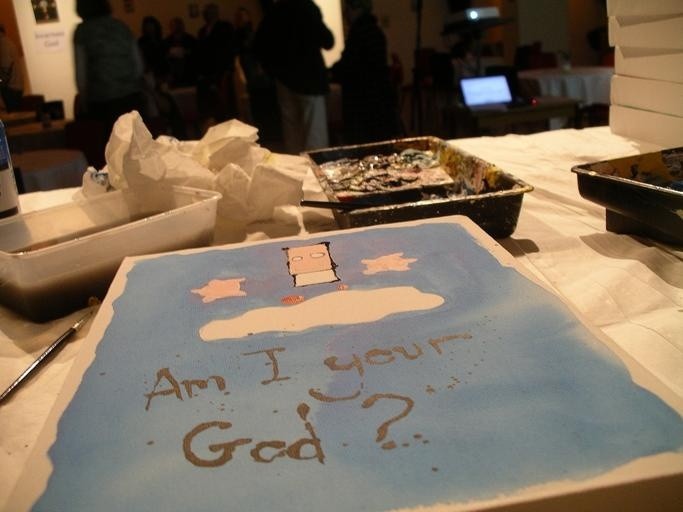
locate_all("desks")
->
[0,123,683,512]
[1,112,87,193]
[518,66,614,128]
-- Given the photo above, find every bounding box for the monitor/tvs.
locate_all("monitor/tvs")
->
[456,74,514,108]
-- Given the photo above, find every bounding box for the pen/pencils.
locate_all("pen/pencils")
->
[0,310,93,405]
[300,201,375,209]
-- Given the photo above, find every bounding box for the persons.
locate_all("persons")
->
[1,24,25,111]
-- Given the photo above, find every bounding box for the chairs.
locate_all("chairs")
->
[11,94,104,167]
